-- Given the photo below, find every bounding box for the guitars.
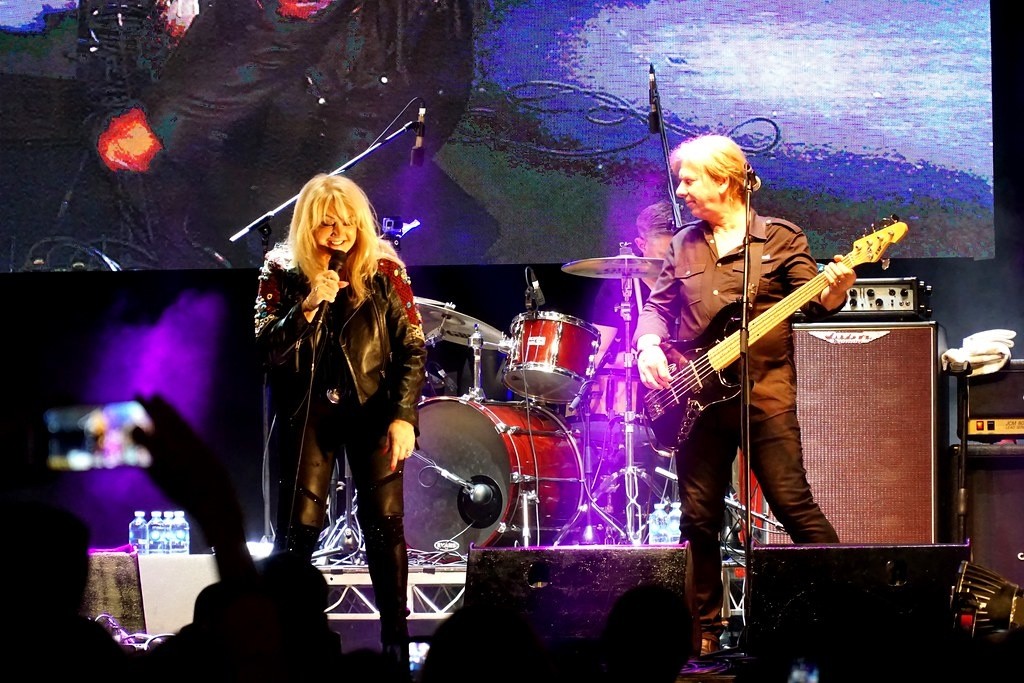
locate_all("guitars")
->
[642,215,912,451]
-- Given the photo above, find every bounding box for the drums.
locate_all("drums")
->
[401,396,585,564]
[565,366,656,446]
[499,308,602,404]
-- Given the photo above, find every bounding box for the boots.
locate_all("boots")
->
[364,515,411,677]
[274,524,320,564]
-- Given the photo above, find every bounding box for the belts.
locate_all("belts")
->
[309,384,358,404]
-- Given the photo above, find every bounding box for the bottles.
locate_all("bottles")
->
[170,511,190,554]
[160,511,174,553]
[128,511,148,554]
[649,504,668,544]
[146,511,165,553]
[667,503,682,544]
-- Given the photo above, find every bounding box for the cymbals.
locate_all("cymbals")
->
[411,302,509,352]
[559,255,668,281]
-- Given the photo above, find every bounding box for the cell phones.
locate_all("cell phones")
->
[42,400,155,472]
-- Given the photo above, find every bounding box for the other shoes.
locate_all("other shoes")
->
[701,632,721,656]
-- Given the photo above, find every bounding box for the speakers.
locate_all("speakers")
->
[950,442,1024,590]
[747,537,973,668]
[762,320,951,548]
[464,540,702,658]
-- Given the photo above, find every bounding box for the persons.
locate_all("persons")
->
[74,0,473,273]
[0,395,1024,683]
[631,135,857,656]
[252,173,428,672]
[592,200,687,530]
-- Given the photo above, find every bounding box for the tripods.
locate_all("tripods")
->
[551,275,671,549]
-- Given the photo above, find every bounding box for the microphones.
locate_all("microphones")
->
[648,64,661,133]
[437,466,492,505]
[531,271,546,305]
[316,250,347,330]
[744,163,761,191]
[410,101,425,167]
